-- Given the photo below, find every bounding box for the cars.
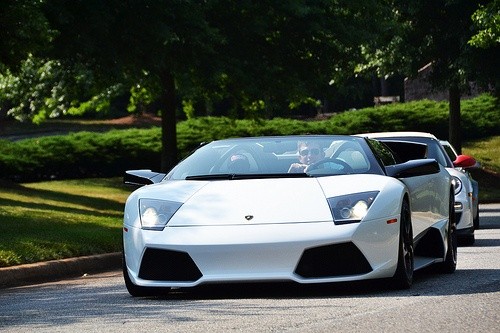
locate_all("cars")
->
[348,127,476,245]
[440,140,480,229]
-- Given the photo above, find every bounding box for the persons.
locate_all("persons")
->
[287,139,324,177]
[227,154,250,177]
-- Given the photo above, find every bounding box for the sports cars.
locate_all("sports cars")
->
[121,134,458,296]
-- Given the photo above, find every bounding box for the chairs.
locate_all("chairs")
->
[209,145,265,173]
[328,140,371,172]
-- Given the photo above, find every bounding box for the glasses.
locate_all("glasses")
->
[299,149,322,156]
